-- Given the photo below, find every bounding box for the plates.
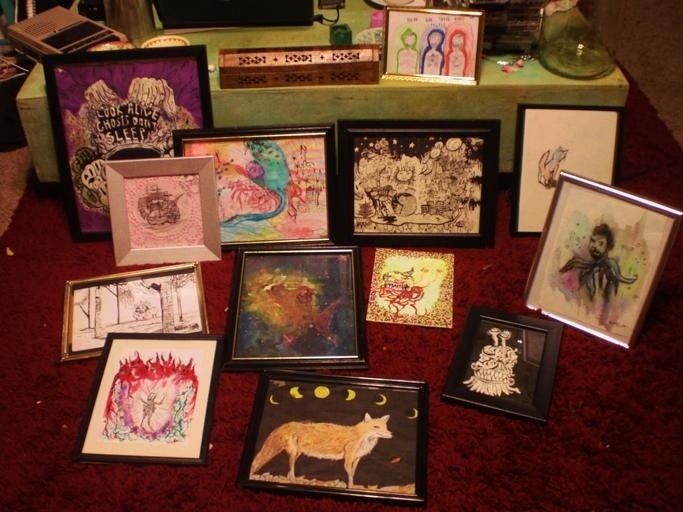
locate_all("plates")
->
[140,34,189,48]
[352,27,383,45]
[88,41,137,52]
[370,0,427,7]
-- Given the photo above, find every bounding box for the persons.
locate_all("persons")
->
[559,224,638,302]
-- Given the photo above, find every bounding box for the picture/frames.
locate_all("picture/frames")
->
[103,153,222,270]
[520,170,682,352]
[58,258,211,365]
[231,367,431,508]
[336,117,503,249]
[437,303,562,426]
[510,102,625,241]
[221,243,370,371]
[173,120,337,253]
[71,329,223,466]
[364,248,456,330]
[377,4,487,87]
[36,41,214,242]
